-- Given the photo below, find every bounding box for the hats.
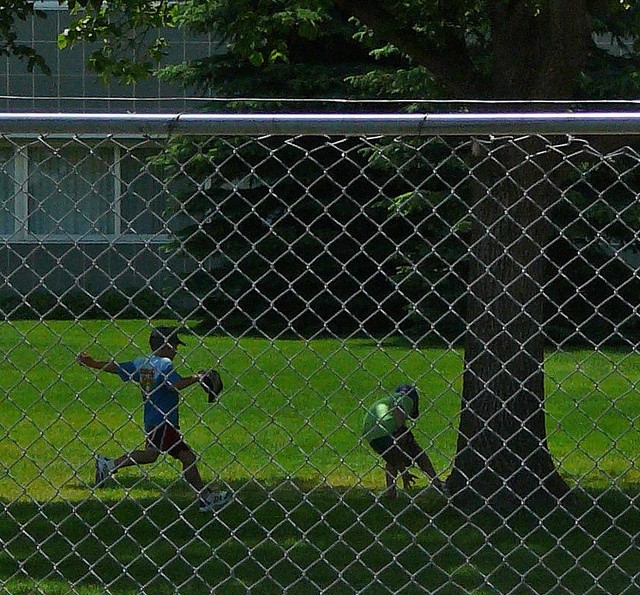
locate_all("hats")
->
[396,384,418,418]
[150,327,186,346]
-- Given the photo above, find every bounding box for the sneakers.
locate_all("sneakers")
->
[96,455,118,488]
[198,488,232,513]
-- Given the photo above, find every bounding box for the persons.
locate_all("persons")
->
[77,325,230,512]
[361,383,444,497]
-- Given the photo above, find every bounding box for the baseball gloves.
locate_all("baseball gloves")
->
[198,367,224,404]
